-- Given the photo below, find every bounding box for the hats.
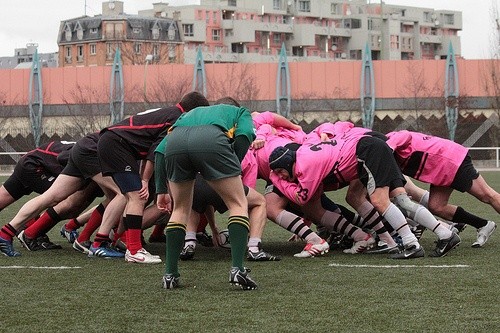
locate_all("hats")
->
[268,146,297,179]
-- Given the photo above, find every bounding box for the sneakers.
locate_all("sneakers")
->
[125,248,161,264]
[115,238,126,252]
[196,231,214,247]
[471,220,497,248]
[227,266,258,289]
[36,235,62,249]
[247,242,281,261]
[148,232,166,243]
[17,230,40,251]
[89,241,124,258]
[60,224,79,243]
[180,242,196,257]
[316,228,467,259]
[162,274,187,289]
[293,239,330,257]
[73,238,92,254]
[0,237,21,256]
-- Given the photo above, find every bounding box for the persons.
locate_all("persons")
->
[154,96,258,290]
[0,92,499,264]
